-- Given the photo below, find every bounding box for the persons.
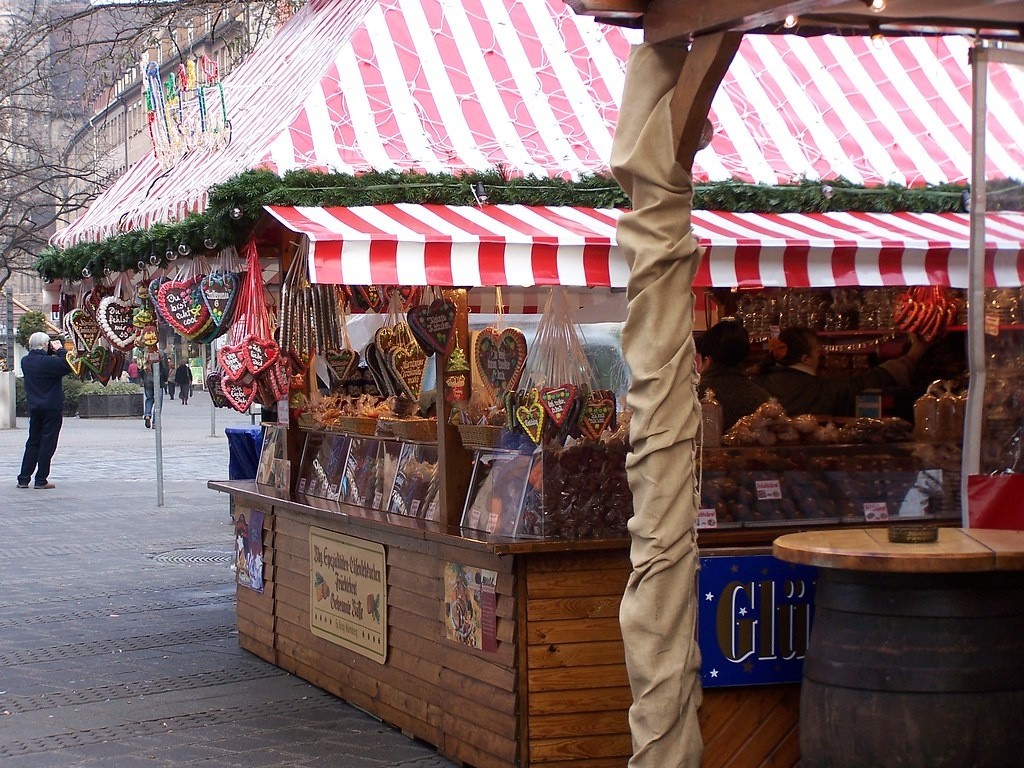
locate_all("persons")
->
[748,325,835,444]
[16,332,70,490]
[865,312,957,440]
[123,347,193,430]
[692,321,777,448]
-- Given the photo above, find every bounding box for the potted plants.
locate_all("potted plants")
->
[78,381,144,416]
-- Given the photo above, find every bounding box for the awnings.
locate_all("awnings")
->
[42,1,1024,540]
[263,206,1024,290]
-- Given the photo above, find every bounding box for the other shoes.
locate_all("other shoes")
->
[185,403,187,405]
[144,416,151,428]
[171,398,174,400]
[17,481,28,488]
[182,402,184,404]
[152,422,162,429]
[34,483,55,489]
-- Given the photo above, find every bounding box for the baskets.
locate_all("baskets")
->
[458,425,502,448]
[297,414,322,429]
[910,471,966,512]
[380,416,438,442]
[339,415,379,437]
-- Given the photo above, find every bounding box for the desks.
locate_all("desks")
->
[774,529,1024,768]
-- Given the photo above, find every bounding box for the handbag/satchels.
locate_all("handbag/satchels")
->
[187,367,189,378]
